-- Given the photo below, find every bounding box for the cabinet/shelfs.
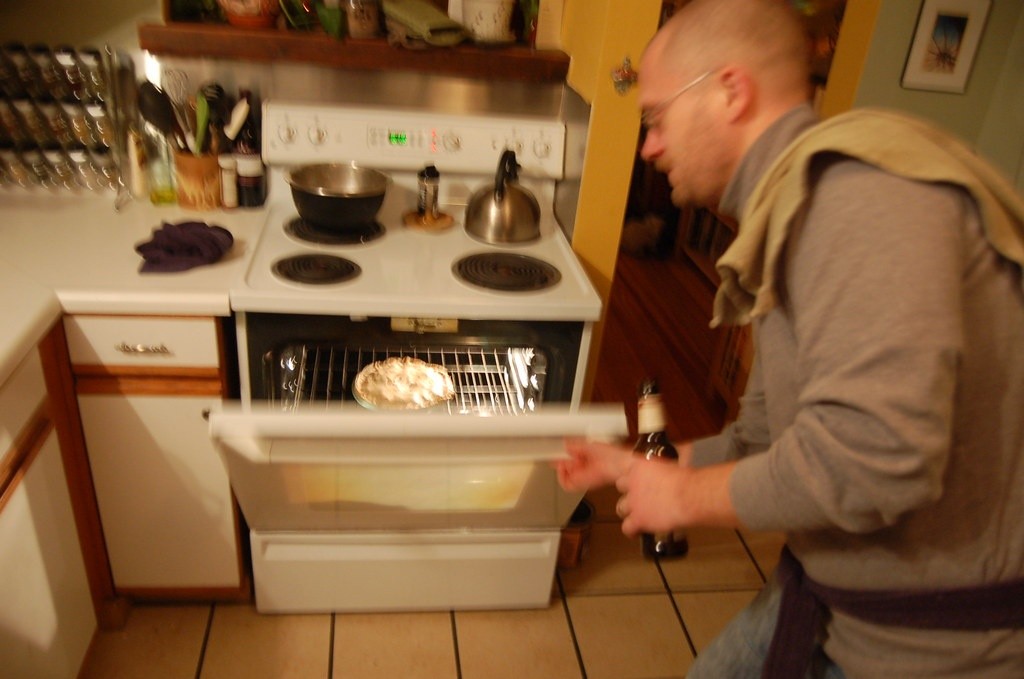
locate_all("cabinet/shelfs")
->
[0,347,104,678]
[60,312,250,608]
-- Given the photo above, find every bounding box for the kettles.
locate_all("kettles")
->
[464,151,542,245]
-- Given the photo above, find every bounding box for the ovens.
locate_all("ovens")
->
[209,312,602,608]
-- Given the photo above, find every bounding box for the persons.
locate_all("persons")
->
[552,0,1024,679]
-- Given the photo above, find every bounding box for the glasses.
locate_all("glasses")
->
[640,64,731,131]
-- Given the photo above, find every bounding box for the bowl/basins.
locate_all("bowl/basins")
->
[285,163,394,230]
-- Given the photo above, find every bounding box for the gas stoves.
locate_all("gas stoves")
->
[234,101,602,324]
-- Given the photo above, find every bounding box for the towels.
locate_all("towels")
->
[708,106,1024,331]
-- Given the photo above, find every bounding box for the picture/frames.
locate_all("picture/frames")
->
[898,1,992,96]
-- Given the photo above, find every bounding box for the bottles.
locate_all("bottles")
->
[0,42,264,210]
[629,379,689,561]
[559,496,595,568]
